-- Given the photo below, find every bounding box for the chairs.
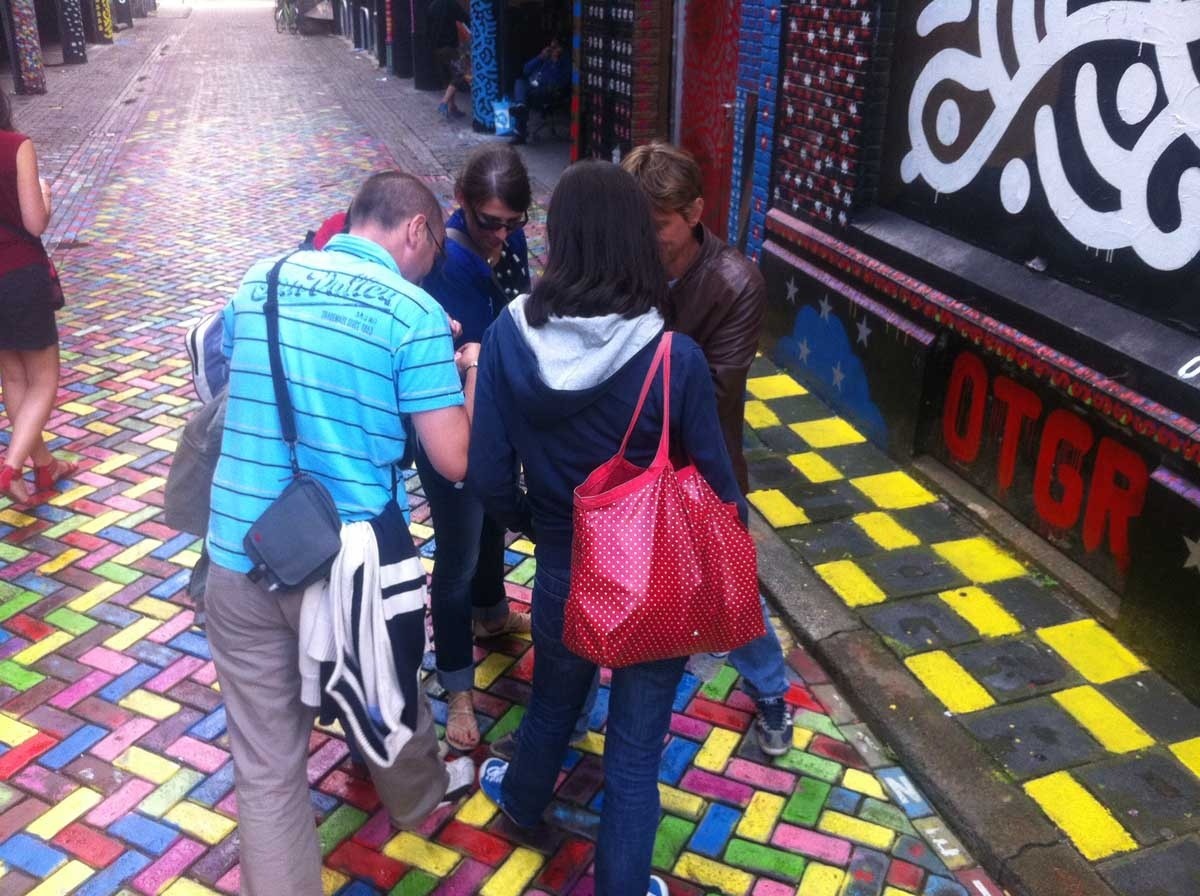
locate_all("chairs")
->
[511,55,574,144]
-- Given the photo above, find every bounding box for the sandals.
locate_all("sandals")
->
[33,459,79,491]
[0,464,36,505]
[472,607,537,636]
[446,693,482,750]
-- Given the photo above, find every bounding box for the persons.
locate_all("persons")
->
[203,171,475,896]
[0,85,80,506]
[295,211,462,783]
[414,143,531,750]
[510,37,573,142]
[428,0,472,118]
[491,144,794,755]
[467,162,749,896]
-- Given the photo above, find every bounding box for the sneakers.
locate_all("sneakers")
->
[645,874,668,896]
[479,754,531,827]
[428,756,476,819]
[754,698,793,756]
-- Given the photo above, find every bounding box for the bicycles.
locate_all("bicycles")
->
[275,0,299,35]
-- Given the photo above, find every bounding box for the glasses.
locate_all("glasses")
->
[408,219,446,262]
[463,187,529,231]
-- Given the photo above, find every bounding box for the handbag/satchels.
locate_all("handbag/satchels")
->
[561,332,769,669]
[243,474,349,594]
